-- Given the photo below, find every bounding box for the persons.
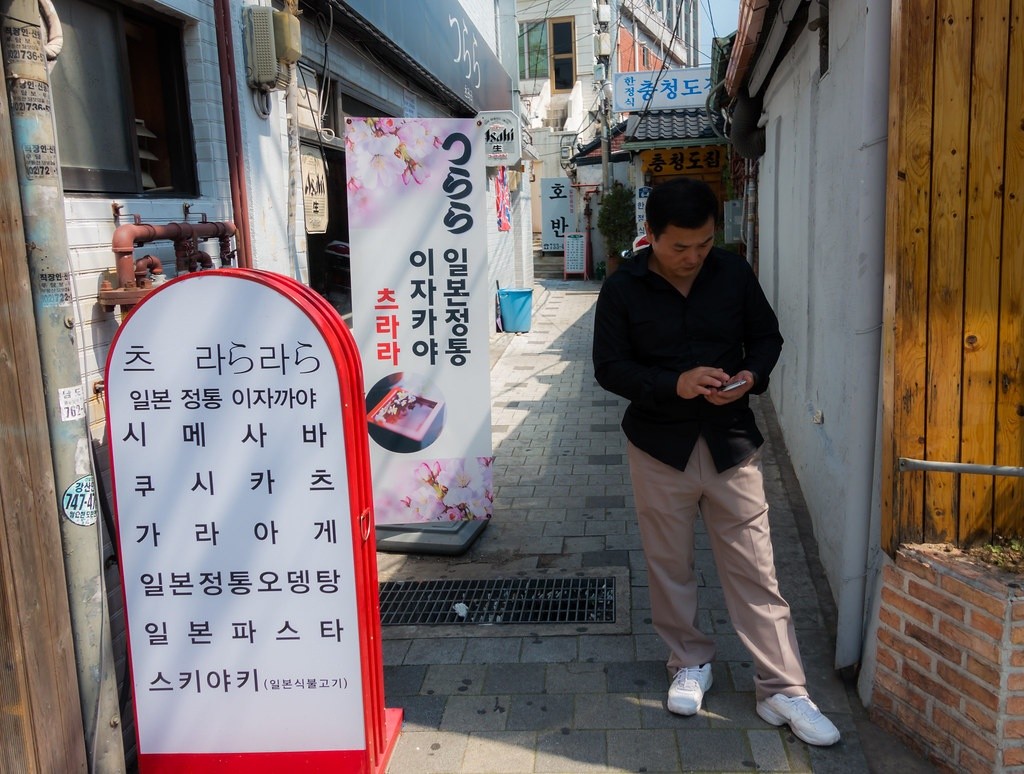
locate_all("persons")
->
[592,178,842,748]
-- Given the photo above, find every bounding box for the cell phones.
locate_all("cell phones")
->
[719,380,748,392]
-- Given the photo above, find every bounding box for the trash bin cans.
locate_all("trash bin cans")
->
[497,288,534,332]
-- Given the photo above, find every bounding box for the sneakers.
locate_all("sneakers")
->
[756,693,840,746]
[667,663,713,715]
